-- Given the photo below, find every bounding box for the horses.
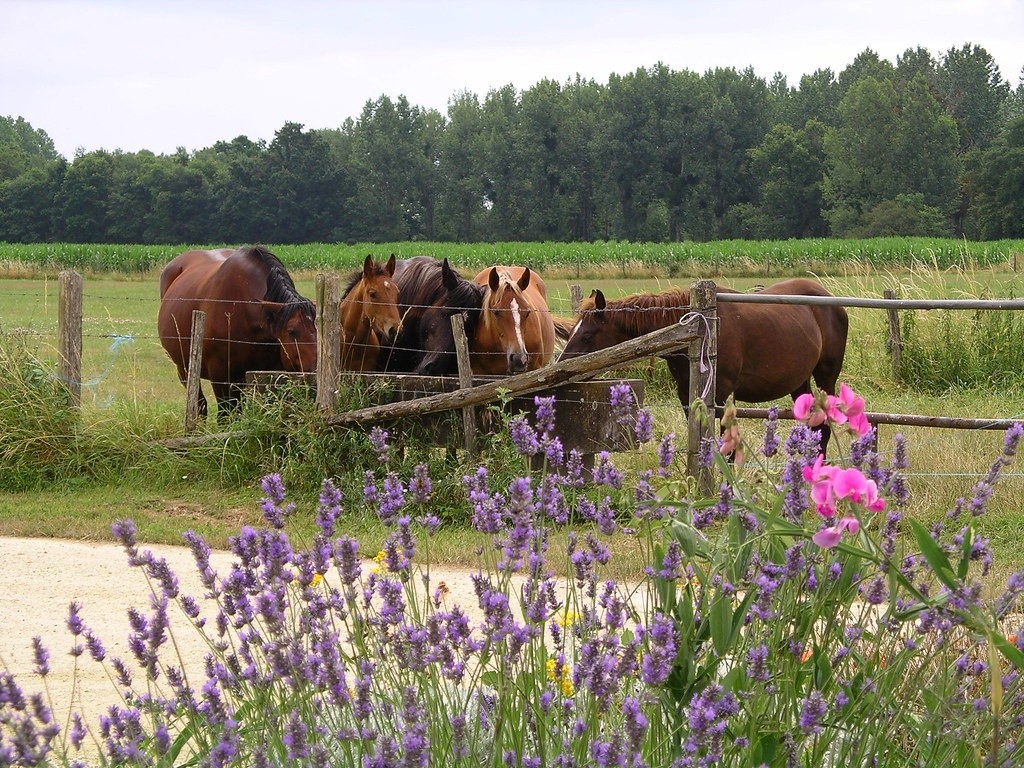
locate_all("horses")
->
[471,265,577,378]
[157,246,318,432]
[553,278,850,468]
[338,252,487,376]
